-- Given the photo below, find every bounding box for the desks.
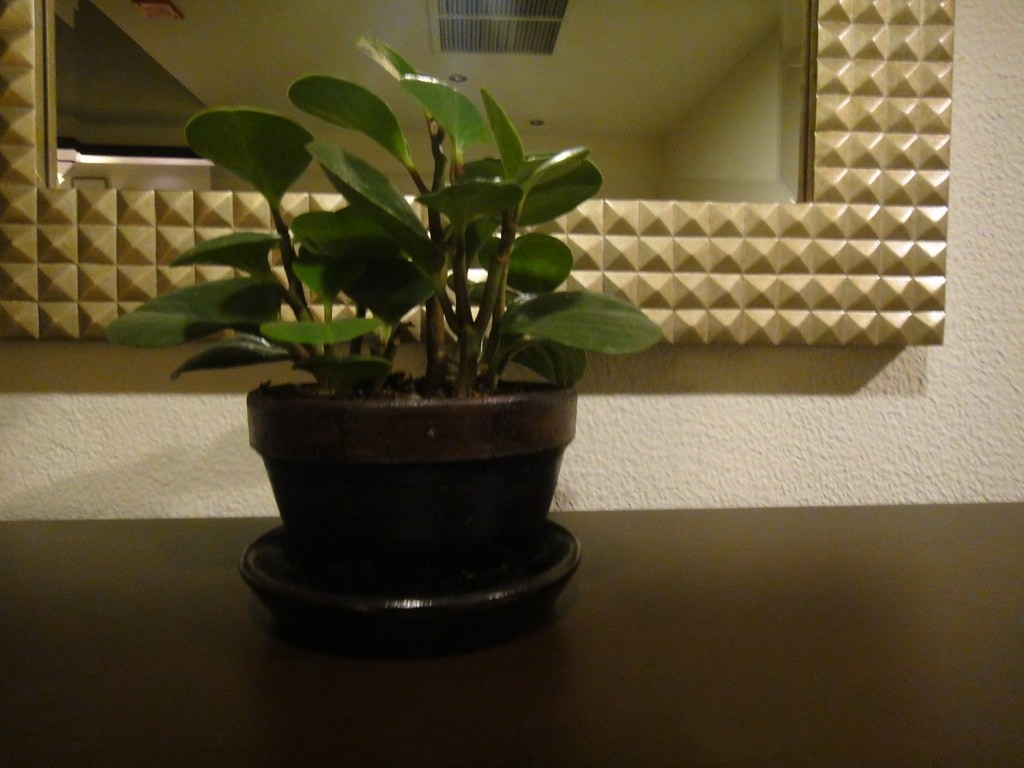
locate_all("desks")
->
[0,501,1024,768]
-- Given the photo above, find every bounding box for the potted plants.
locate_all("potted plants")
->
[101,33,663,665]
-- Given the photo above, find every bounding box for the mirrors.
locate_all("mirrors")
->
[1,0,957,352]
